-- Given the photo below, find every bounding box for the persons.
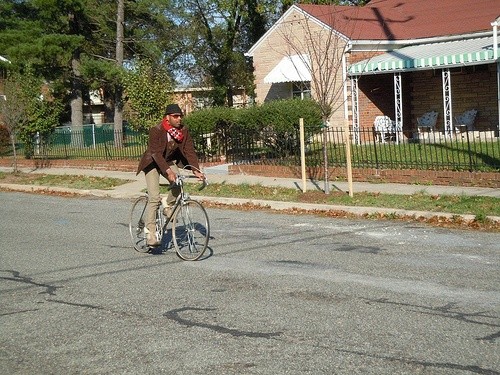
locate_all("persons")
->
[138,105,204,248]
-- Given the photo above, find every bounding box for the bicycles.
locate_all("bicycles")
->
[128,165,211,262]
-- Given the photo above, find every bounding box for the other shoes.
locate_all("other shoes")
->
[144,224,160,245]
[161,204,178,223]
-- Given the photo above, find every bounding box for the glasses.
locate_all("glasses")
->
[168,114,184,119]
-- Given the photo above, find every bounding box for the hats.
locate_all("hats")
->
[163,104,183,115]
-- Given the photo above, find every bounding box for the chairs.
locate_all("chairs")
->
[454,109,477,133]
[373,116,396,142]
[417,111,438,134]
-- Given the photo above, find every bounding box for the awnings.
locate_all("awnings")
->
[350,38,500,134]
[264,56,312,84]
